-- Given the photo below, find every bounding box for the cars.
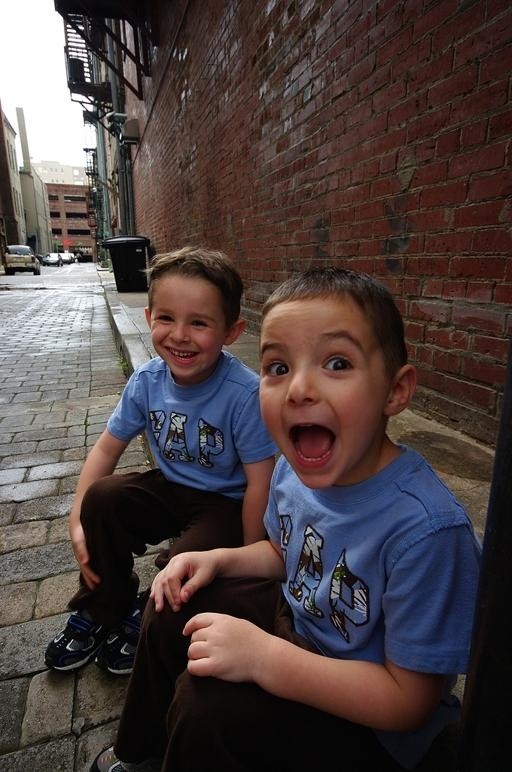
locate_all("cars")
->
[41,252,76,266]
[3,244,41,275]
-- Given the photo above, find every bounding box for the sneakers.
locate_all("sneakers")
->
[45,606,115,675]
[95,585,151,675]
[89,743,162,771]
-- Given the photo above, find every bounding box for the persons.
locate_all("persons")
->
[41,245,281,677]
[85,269,485,772]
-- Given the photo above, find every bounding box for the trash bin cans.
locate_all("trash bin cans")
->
[101,236,150,293]
